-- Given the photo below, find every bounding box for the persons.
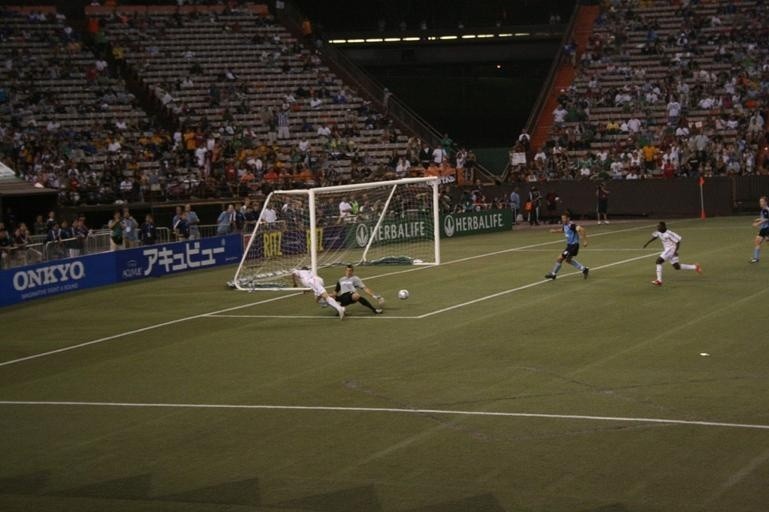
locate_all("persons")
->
[325,264,383,315]
[0,1,768,286]
[290,266,346,321]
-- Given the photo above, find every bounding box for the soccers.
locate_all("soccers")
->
[399,290,409,300]
